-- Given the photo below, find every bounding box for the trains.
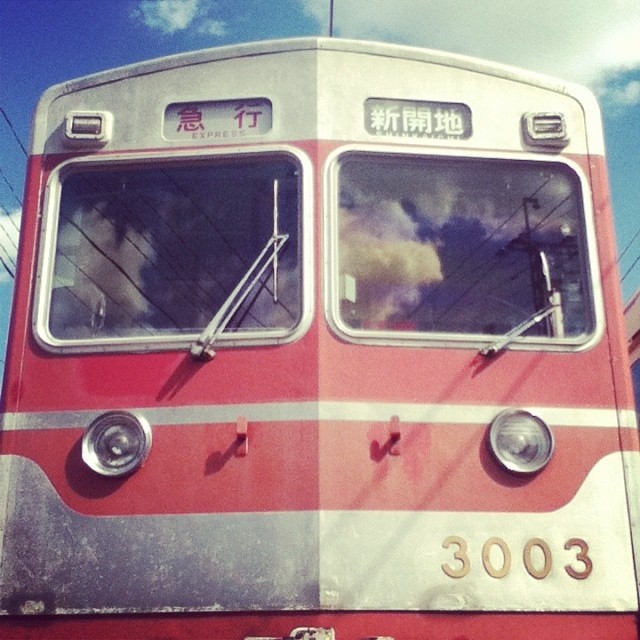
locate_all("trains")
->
[1,36,640,635]
